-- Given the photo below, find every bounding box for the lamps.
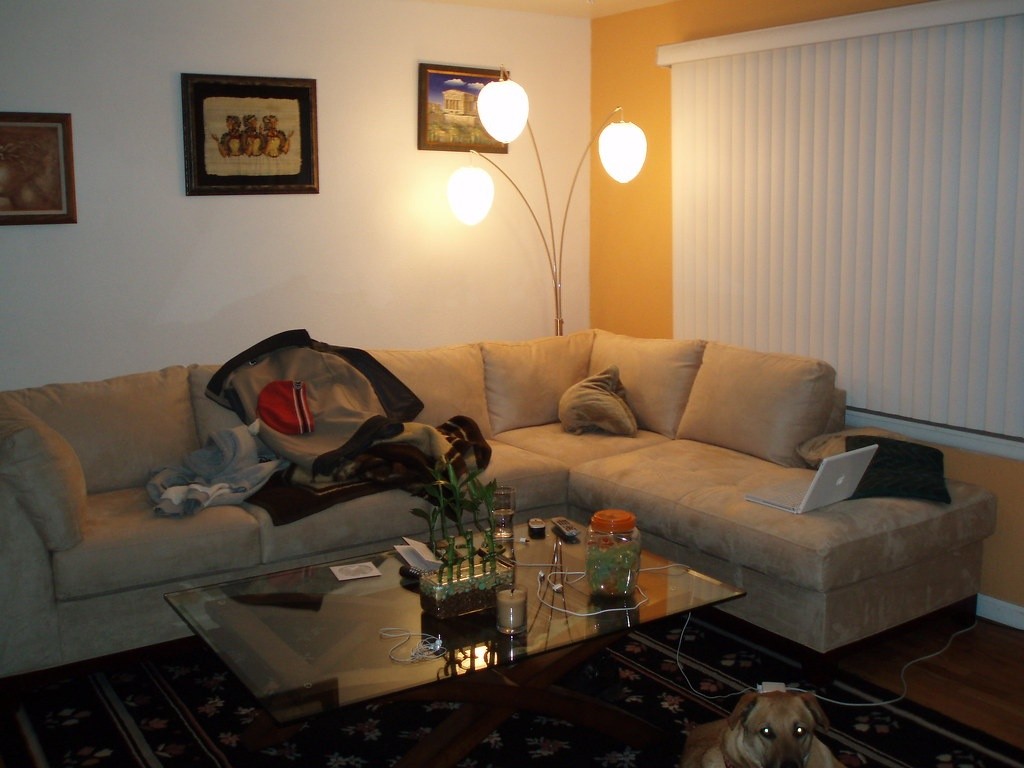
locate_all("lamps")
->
[445,65,648,336]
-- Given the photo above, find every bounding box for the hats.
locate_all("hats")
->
[256,380,315,435]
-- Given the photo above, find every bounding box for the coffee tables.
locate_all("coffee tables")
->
[164,517,746,768]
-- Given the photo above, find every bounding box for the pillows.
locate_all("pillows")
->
[843,435,950,505]
[558,364,638,438]
[795,428,940,470]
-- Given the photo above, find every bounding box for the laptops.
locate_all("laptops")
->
[744,444,879,514]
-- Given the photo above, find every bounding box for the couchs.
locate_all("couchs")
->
[1,328,998,679]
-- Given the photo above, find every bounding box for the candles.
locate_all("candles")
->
[497,584,526,629]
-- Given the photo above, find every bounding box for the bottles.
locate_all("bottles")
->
[584,508,642,602]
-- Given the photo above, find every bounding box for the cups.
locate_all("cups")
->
[493,484,517,539]
[498,585,529,631]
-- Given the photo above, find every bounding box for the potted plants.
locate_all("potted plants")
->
[409,459,513,620]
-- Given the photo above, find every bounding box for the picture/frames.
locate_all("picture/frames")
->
[0,111,77,226]
[180,73,319,197]
[417,62,510,154]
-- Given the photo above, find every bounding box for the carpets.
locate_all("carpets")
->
[0,607,1024,768]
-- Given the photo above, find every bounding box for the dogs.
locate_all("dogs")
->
[682,691,848,768]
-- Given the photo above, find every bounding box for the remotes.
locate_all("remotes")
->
[551,517,581,537]
[528,518,545,531]
[399,565,431,580]
